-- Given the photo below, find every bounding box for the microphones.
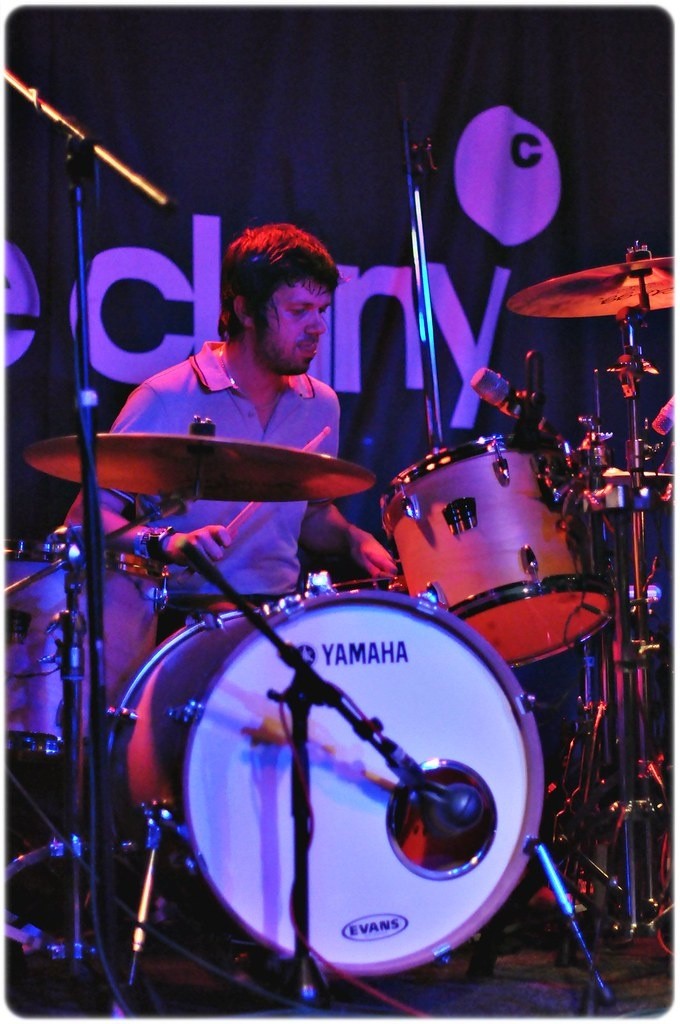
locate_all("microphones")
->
[471,367,547,437]
[432,784,483,834]
[652,396,674,436]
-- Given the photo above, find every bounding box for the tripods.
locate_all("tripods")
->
[454,304,674,984]
[6,452,216,1022]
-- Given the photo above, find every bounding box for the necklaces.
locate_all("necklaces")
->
[219,345,282,409]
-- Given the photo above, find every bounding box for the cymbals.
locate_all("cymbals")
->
[21,431,379,504]
[598,466,674,485]
[505,256,675,319]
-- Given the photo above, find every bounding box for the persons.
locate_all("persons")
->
[64,222,397,966]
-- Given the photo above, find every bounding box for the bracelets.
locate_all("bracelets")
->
[134,527,159,560]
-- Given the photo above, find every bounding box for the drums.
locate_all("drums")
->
[5,530,164,766]
[378,430,616,670]
[106,587,548,983]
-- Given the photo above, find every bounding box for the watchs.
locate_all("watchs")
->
[147,526,176,566]
